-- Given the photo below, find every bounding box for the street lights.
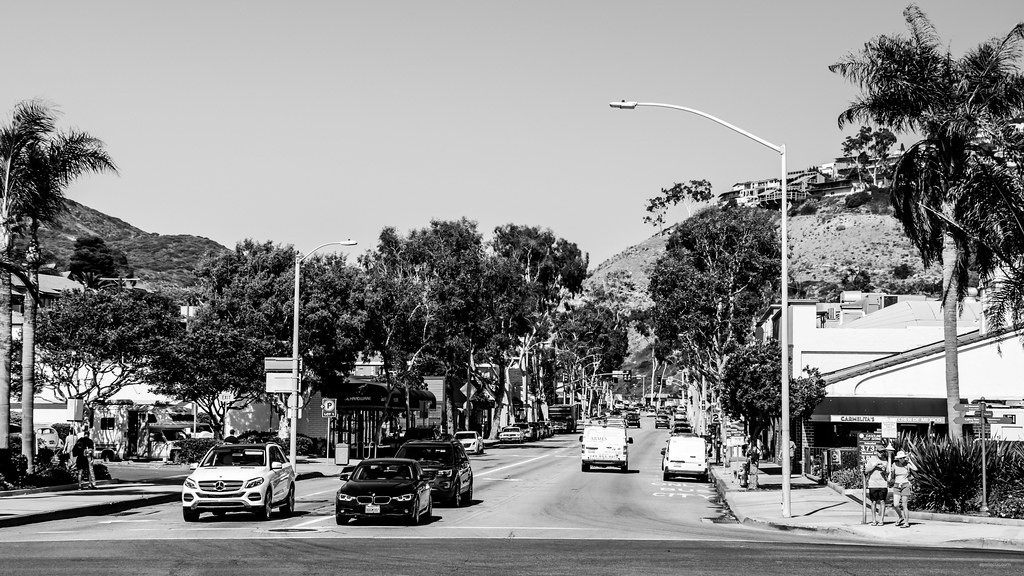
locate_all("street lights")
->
[289,239,357,473]
[609,100,792,518]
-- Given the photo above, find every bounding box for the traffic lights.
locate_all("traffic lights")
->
[663,380,666,388]
[657,375,662,385]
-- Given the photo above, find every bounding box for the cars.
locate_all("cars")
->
[498,421,555,443]
[614,399,645,428]
[335,459,434,525]
[454,432,485,454]
[646,406,709,482]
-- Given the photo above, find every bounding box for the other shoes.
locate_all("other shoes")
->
[871,522,877,526]
[900,524,910,528]
[895,518,904,526]
[878,521,884,526]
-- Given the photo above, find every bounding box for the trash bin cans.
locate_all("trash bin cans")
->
[334,443,349,465]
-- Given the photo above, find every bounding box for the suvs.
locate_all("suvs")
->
[392,439,474,507]
[135,422,214,462]
[183,442,295,522]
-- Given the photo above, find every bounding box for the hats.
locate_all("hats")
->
[895,451,907,459]
[877,445,886,451]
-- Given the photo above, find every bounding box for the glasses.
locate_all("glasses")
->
[900,457,905,459]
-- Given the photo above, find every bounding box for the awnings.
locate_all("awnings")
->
[450,368,498,402]
[338,382,436,412]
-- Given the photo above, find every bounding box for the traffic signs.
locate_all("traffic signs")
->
[953,403,981,413]
[953,417,980,425]
[986,417,1012,424]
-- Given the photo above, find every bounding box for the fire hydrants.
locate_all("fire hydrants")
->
[738,465,748,486]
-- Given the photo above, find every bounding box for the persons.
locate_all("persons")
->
[888,450,918,528]
[778,433,797,479]
[63,430,78,469]
[864,445,888,526]
[223,429,241,444]
[748,438,764,468]
[72,432,98,491]
[277,414,289,441]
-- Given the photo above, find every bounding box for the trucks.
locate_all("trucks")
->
[579,418,634,473]
[549,396,579,432]
